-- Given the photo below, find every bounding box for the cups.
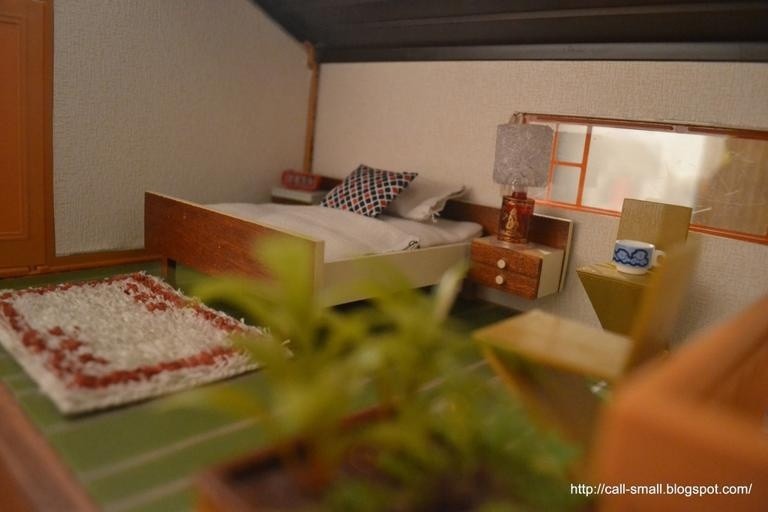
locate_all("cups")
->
[612,239,668,276]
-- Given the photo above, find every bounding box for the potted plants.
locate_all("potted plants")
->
[186,222,598,512]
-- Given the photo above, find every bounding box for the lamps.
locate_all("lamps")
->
[494,123,555,247]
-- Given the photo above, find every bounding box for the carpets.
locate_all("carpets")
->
[1,271,295,418]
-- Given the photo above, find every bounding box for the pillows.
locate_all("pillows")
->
[317,164,469,226]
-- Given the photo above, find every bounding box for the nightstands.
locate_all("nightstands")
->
[469,233,564,299]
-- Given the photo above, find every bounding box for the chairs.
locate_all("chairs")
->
[466,246,699,463]
[580,200,694,330]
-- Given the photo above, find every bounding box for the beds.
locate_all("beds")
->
[145,181,573,351]
